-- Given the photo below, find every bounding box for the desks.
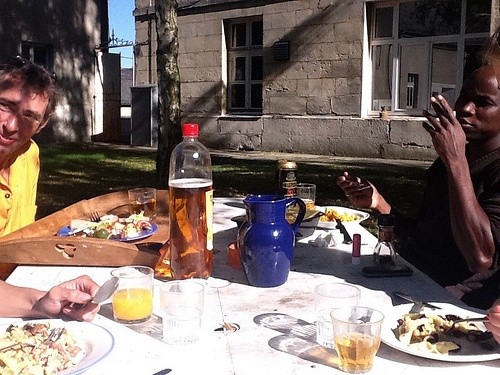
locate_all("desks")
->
[0,198,500,375]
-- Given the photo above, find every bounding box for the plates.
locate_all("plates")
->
[0,317,114,375]
[316,205,371,229]
[56,218,159,242]
[371,301,500,364]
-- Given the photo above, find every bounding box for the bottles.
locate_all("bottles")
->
[372,215,396,267]
[167,124,213,280]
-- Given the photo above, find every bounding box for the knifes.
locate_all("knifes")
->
[393,291,440,310]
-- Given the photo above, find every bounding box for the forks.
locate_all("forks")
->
[89,210,100,223]
[79,276,119,311]
[45,326,70,347]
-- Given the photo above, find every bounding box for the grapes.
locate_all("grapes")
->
[96,229,109,240]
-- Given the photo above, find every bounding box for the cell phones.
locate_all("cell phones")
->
[363,265,414,277]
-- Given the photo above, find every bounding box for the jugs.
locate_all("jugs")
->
[237,194,305,288]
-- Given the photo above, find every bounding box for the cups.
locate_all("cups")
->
[313,283,360,350]
[328,305,384,374]
[160,279,205,345]
[127,187,156,218]
[112,265,156,324]
[295,183,317,214]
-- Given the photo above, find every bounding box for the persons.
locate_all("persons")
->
[337,50,500,310]
[0,274,101,322]
[483,298,500,344]
[0,58,54,236]
